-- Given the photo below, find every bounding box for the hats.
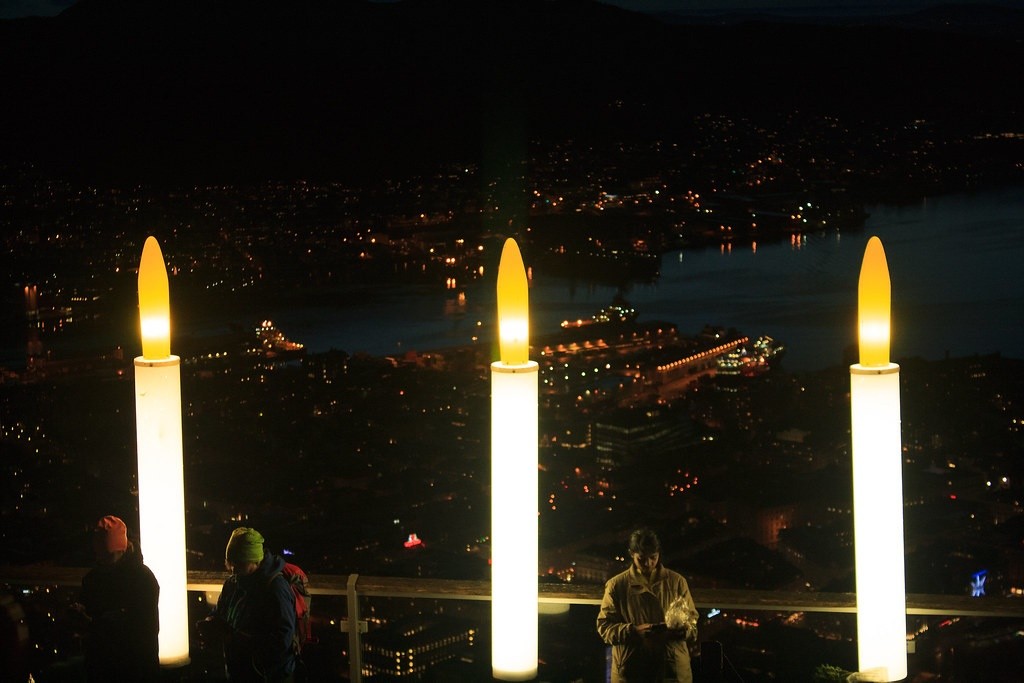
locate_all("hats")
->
[225,527,266,564]
[91,515,127,551]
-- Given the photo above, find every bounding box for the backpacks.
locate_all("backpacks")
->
[283,561,319,655]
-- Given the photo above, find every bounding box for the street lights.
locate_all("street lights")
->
[128,234,194,666]
[843,234,911,682]
[484,238,544,681]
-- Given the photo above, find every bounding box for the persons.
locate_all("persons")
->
[196,526,311,683]
[596,528,700,683]
[67,514,160,683]
